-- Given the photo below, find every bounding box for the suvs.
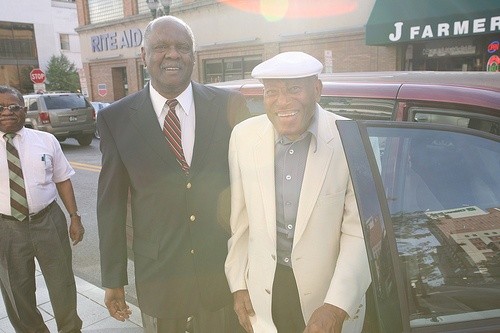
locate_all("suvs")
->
[21,90,96,146]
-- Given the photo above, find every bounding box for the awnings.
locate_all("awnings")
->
[365,0,500,45]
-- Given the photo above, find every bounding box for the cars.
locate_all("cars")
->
[199,67,500,333]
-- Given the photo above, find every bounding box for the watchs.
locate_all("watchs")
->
[70,210,81,218]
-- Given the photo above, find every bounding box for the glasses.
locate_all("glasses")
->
[0,105,24,112]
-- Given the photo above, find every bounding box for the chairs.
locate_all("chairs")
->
[401,148,500,287]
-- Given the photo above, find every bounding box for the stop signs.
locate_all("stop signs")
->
[30,69,46,84]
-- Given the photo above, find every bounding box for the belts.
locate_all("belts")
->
[0,201,55,222]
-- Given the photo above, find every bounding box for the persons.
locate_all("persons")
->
[0,86,85,333]
[97,14,253,333]
[225,51,386,333]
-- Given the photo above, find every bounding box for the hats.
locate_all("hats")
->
[251,51,324,83]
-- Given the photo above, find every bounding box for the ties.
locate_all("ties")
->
[3,132,29,223]
[162,99,190,176]
